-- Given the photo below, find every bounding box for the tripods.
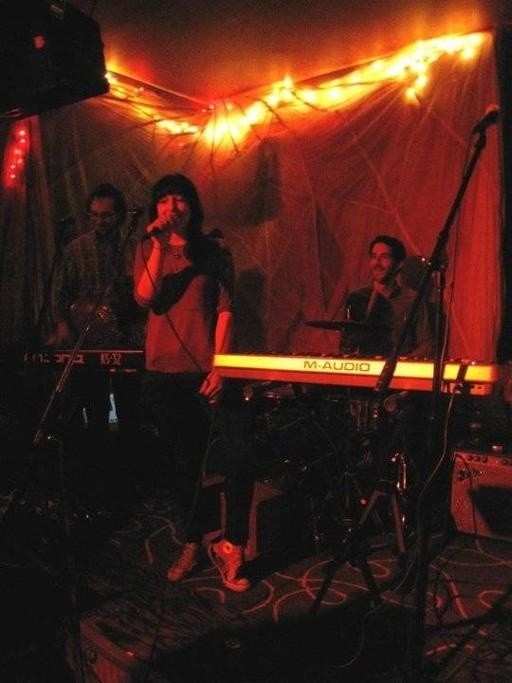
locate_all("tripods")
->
[303,133,489,617]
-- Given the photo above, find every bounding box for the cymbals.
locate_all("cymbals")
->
[305,318,380,336]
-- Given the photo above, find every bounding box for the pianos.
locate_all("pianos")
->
[5,350,144,373]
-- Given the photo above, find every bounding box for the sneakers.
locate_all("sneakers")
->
[167,542,203,582]
[208,537,253,593]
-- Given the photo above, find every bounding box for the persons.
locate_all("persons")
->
[339,235,443,357]
[134,175,251,593]
[52,184,145,435]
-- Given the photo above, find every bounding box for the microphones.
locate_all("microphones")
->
[141,212,183,242]
[467,102,505,132]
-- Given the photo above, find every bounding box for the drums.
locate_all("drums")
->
[242,380,307,429]
